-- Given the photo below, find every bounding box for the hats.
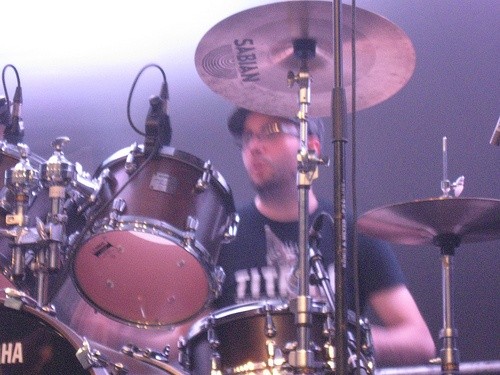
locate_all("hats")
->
[227,105,324,142]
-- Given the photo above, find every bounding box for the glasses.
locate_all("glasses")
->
[234,121,300,147]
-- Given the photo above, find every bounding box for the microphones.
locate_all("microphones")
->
[289,215,323,293]
[6,87,25,146]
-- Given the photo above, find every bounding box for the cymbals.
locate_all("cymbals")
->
[194,0,416,120]
[355,196,500,246]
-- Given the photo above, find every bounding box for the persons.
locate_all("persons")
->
[215,105,436,366]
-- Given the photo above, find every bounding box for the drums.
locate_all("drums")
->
[177,297,375,375]
[0,296,98,374]
[0,142,21,190]
[67,144,240,332]
